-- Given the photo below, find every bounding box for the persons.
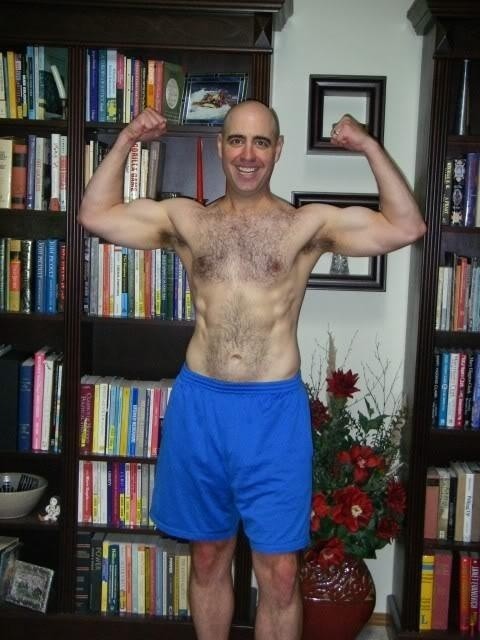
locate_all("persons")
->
[74,92,429,639]
[39,497,61,521]
[16,575,42,600]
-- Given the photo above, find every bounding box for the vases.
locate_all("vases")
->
[297,549,377,640]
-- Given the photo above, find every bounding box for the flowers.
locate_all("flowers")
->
[302,323,408,569]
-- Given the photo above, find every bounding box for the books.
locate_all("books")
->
[406,47,479,631]
[76,375,177,527]
[1,47,198,321]
[76,532,234,617]
[1,343,64,453]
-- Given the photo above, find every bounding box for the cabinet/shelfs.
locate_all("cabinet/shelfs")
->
[0,0,285,639]
[386,0,480,640]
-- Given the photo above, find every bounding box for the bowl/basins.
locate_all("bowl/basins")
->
[0,473,49,519]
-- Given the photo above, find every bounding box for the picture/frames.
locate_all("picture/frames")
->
[179,72,249,128]
[292,191,388,292]
[308,72,387,153]
